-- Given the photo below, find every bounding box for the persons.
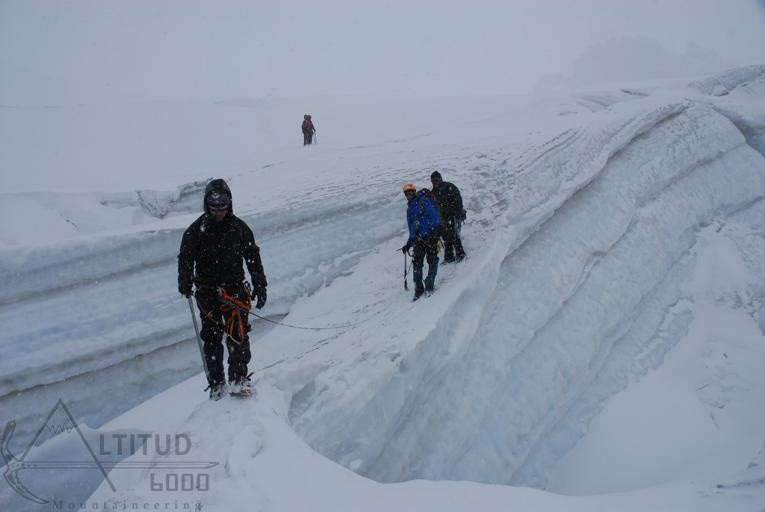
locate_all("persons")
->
[428,170,467,266]
[301,114,310,146]
[401,182,443,302]
[303,115,316,146]
[177,178,268,400]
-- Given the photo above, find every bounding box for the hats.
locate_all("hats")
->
[208,192,230,206]
[431,172,441,182]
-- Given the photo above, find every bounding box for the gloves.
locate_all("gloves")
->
[251,287,267,309]
[403,242,412,253]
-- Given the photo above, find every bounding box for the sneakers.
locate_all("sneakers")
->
[413,288,423,302]
[456,253,466,264]
[210,381,225,401]
[441,256,455,265]
[229,378,253,397]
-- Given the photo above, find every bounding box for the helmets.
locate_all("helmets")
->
[404,184,417,192]
